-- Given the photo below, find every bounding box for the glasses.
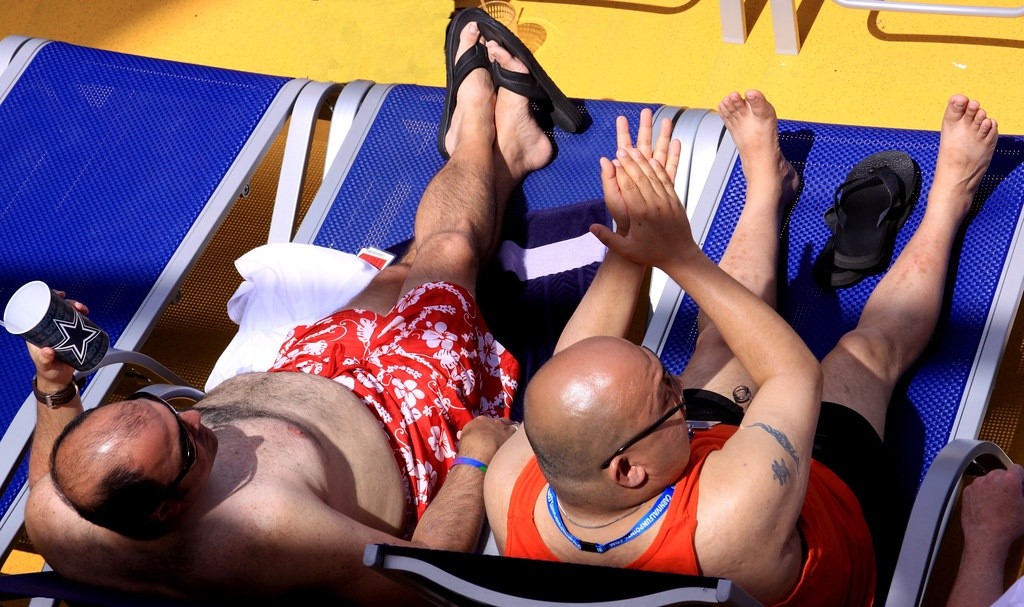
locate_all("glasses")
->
[122,392,195,510]
[601,346,687,469]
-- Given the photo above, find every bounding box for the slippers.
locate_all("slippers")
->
[438,7,494,160]
[813,150,917,287]
[478,16,583,133]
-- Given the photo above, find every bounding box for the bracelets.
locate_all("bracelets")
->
[33,372,87,408]
[448,457,487,471]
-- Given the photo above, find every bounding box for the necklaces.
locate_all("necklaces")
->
[557,494,644,528]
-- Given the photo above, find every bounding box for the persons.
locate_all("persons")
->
[24,5,587,607]
[482,89,999,607]
[945,463,1024,607]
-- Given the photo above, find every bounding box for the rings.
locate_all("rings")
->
[510,425,519,430]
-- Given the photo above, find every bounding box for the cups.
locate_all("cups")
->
[3,280,110,373]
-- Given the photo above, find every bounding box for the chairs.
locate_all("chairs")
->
[0,0,1024,607]
